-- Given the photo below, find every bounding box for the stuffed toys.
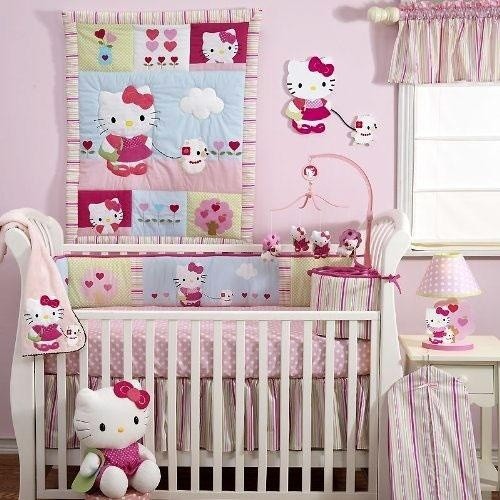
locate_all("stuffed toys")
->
[260,236,280,260]
[336,230,361,257]
[425,307,453,345]
[290,225,311,252]
[71,379,162,499]
[310,230,331,258]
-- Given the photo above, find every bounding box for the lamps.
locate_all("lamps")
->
[416,251,481,349]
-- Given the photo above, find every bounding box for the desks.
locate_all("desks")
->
[399,334,500,500]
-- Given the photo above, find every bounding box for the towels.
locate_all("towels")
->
[0,207,86,357]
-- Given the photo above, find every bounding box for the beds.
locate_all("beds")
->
[4,207,412,500]
